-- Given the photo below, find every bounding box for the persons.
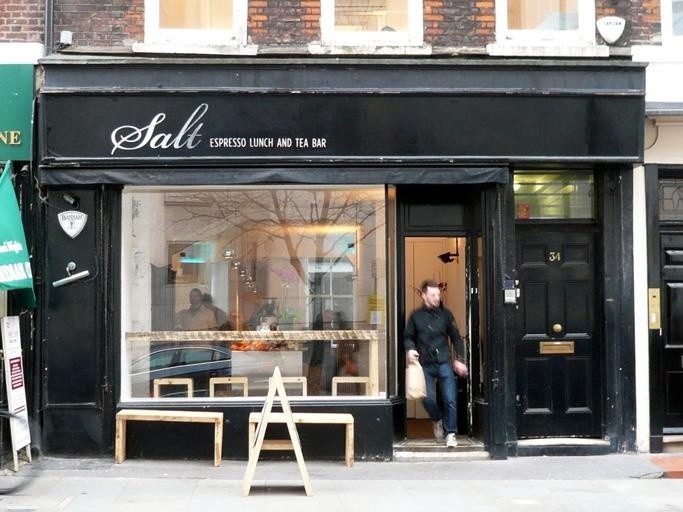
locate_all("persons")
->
[218,287,361,396]
[201,293,225,330]
[171,287,217,367]
[406,278,468,447]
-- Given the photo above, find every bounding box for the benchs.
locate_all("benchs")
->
[248,411,354,468]
[115,408,224,468]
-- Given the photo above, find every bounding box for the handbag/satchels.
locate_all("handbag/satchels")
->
[405,365,427,401]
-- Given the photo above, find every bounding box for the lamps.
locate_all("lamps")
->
[223,192,259,294]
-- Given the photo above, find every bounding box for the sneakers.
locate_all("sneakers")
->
[446,433,457,448]
[433,419,445,438]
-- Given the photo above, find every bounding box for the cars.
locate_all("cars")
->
[129,345,236,397]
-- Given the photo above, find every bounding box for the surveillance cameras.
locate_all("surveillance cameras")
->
[63,194,76,205]
[20,164,30,177]
